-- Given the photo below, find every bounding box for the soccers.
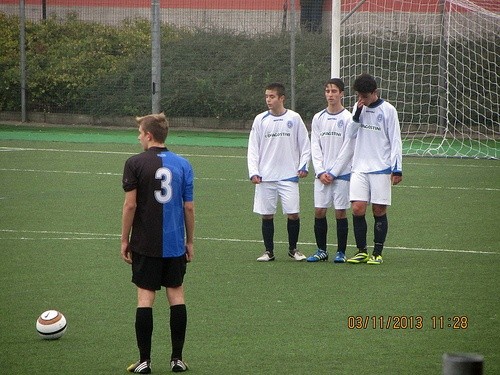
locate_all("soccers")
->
[35,309,68,340]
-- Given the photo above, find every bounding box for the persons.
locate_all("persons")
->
[347,74,402,265]
[308,78,355,262]
[120,113,195,375]
[248,83,311,262]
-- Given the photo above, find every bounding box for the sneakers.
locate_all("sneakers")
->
[307,248,328,261]
[347,252,369,263]
[256,251,275,262]
[170,359,186,372]
[287,249,306,261]
[127,359,152,374]
[334,249,346,263]
[367,254,383,264]
